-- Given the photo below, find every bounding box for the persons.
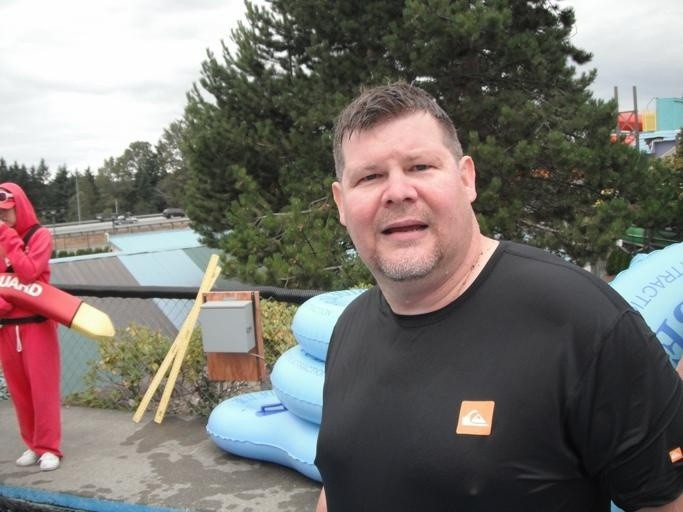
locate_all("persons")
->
[0,182,63,471]
[312,82,683,511]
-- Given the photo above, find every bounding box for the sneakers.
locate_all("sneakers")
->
[15,449,59,471]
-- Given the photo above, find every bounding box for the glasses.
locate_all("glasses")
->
[0,192,13,202]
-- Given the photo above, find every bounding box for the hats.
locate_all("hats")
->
[0,188,16,209]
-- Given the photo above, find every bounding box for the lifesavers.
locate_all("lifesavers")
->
[292,290,367,361]
[611,242,682,512]
[270,345,326,423]
[206,388,321,485]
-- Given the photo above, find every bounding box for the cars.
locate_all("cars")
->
[94,206,186,228]
